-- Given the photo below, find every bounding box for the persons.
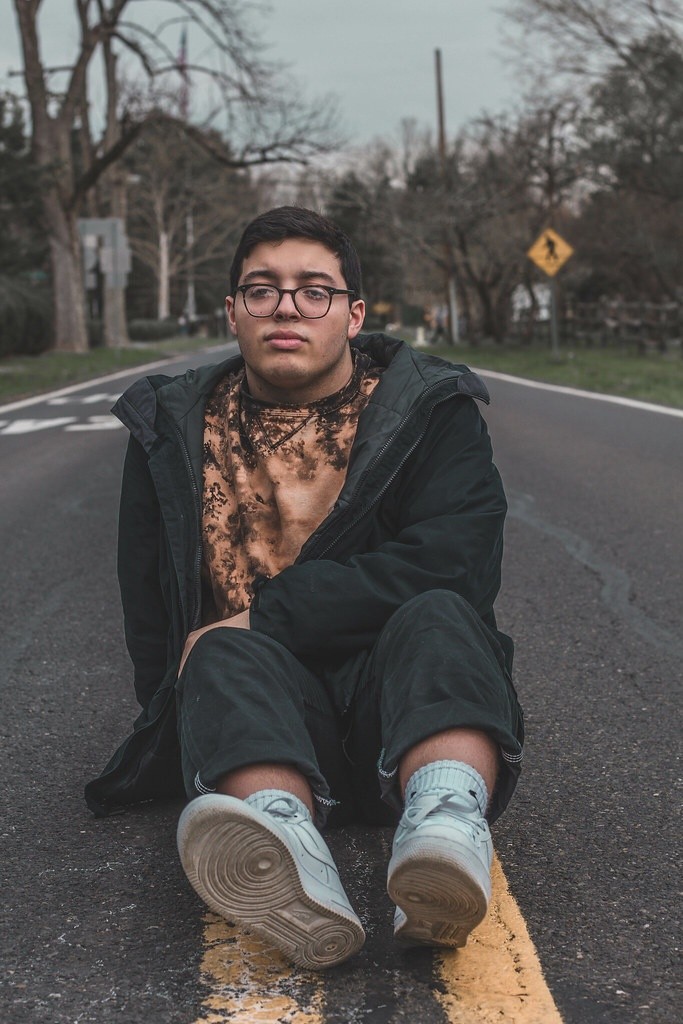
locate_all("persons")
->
[85,207,525,971]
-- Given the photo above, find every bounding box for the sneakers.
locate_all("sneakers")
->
[176,791,365,969]
[387,792,494,949]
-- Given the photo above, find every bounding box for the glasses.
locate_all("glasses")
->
[231,285,356,320]
